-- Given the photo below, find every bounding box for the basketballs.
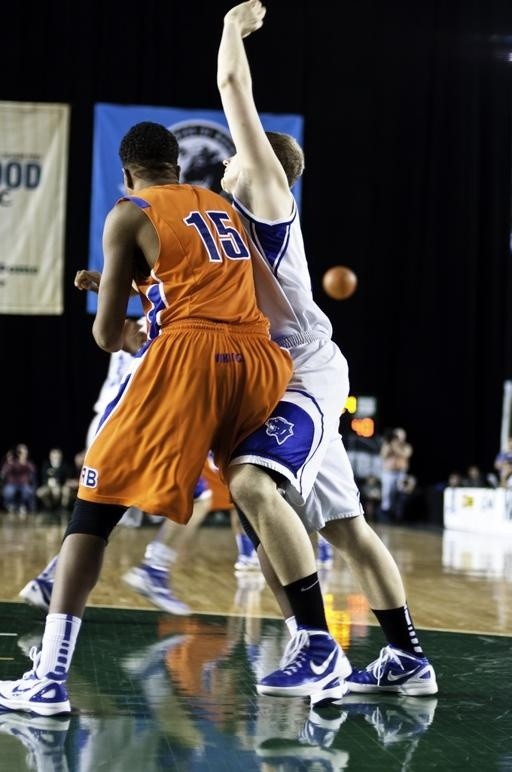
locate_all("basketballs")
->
[324,267,357,299]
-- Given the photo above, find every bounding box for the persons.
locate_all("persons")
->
[144,449,261,569]
[316,531,333,570]
[0,121,349,717]
[74,0,439,698]
[19,316,213,615]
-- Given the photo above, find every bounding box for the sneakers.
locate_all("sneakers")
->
[20,578,53,606]
[122,562,191,618]
[0,717,70,749]
[235,552,265,570]
[255,697,437,771]
[0,672,70,715]
[120,634,193,684]
[18,626,44,661]
[255,632,438,706]
[318,543,334,570]
